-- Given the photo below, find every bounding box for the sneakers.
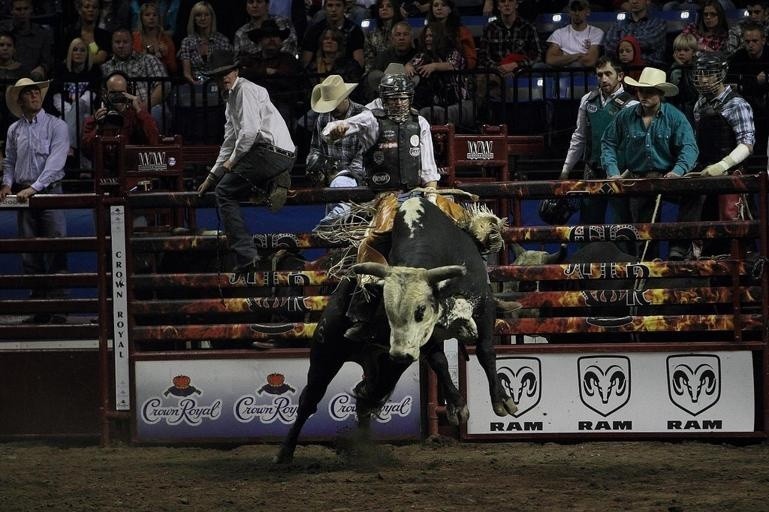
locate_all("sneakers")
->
[234,260,261,272]
[344,320,376,341]
[267,173,290,214]
[496,297,521,315]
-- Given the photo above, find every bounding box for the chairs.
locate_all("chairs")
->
[408,6,750,113]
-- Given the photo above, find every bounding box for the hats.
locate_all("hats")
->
[6,78,51,118]
[201,49,239,77]
[311,74,359,114]
[625,67,679,97]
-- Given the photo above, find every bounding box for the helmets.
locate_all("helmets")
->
[693,54,729,95]
[379,72,414,123]
[538,198,575,225]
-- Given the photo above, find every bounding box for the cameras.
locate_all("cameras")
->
[110,92,125,105]
[146,45,155,57]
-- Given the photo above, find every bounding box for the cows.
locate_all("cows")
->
[269,194,518,472]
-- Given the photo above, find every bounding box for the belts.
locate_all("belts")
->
[263,143,295,158]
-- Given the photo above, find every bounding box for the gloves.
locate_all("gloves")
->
[701,155,737,177]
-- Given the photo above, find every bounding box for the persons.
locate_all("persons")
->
[0,77,71,330]
[0,0,769,109]
[302,73,379,232]
[192,49,299,274]
[78,68,184,227]
[689,51,757,257]
[599,67,700,259]
[561,54,640,255]
[319,62,524,341]
